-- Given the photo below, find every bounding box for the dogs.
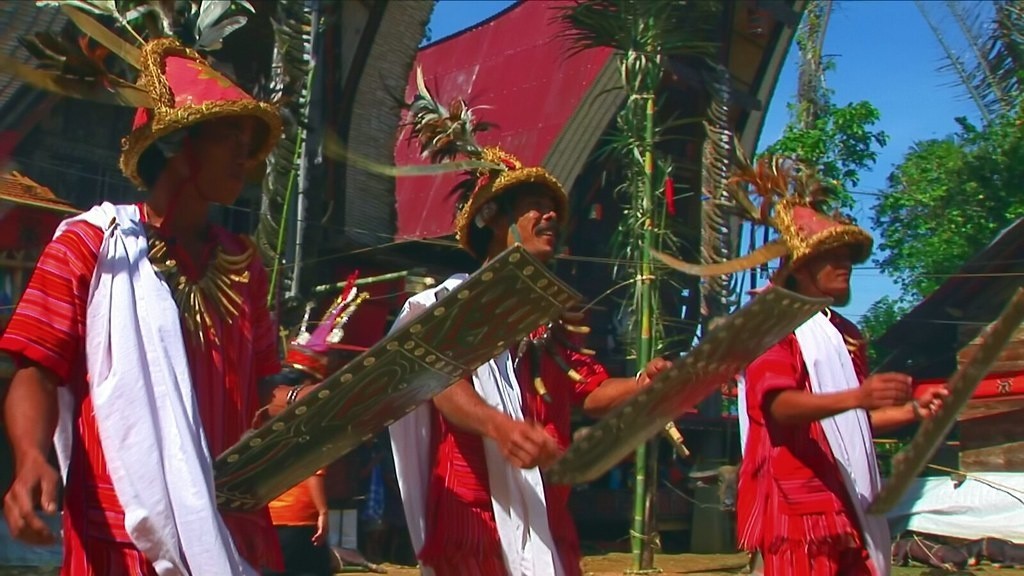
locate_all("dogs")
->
[890,538,967,573]
[959,536,1024,570]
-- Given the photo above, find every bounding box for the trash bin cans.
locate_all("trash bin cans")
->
[689,470,737,554]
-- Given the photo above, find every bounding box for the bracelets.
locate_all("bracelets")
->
[913,399,920,419]
[635,367,644,386]
[286,385,305,407]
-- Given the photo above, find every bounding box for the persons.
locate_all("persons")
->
[734,199,949,576]
[0,38,322,576]
[259,346,331,576]
[415,147,671,576]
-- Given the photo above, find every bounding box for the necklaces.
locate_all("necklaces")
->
[143,197,253,350]
[514,319,596,404]
[825,308,831,321]
